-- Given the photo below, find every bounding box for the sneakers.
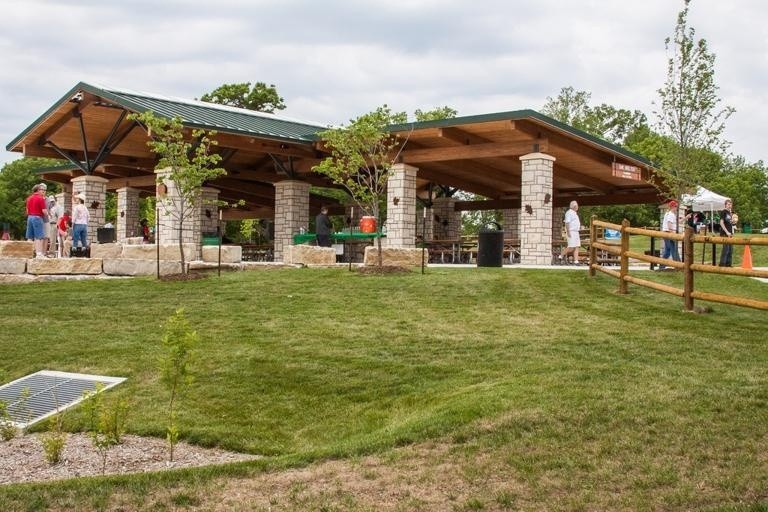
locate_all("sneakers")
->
[37,253,46,258]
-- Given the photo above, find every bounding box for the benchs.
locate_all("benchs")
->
[425,248,516,263]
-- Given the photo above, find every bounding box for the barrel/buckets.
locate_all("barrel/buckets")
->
[359,215,376,233]
[97,228,113,244]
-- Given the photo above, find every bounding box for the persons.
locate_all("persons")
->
[104,218,115,228]
[140,219,150,244]
[559,201,582,266]
[316,206,333,246]
[25,183,90,258]
[658,200,739,271]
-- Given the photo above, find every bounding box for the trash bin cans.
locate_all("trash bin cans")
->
[744,224,752,234]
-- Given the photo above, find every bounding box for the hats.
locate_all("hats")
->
[74,194,85,201]
[669,201,678,207]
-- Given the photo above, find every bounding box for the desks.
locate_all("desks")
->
[425,238,521,247]
